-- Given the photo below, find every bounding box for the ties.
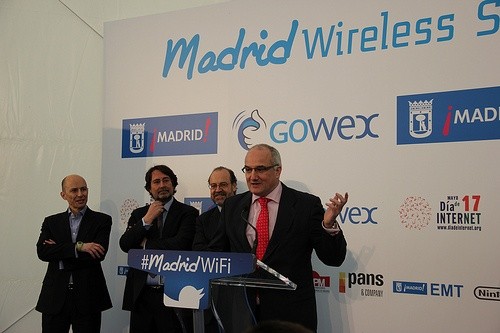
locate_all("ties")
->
[256,197,274,305]
[158,208,166,241]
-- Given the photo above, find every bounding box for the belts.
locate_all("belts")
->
[150,284,161,289]
[67,282,75,290]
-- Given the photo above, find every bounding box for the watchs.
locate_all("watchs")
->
[324,221,338,229]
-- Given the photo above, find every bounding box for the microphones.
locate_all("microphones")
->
[240,205,258,254]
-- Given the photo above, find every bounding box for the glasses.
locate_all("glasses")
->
[242,164,278,173]
[209,182,234,188]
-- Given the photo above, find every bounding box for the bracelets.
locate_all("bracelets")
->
[77,240,83,251]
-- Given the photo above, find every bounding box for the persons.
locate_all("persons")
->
[121,166,198,333]
[34,174,113,333]
[207,144,349,333]
[191,167,237,333]
